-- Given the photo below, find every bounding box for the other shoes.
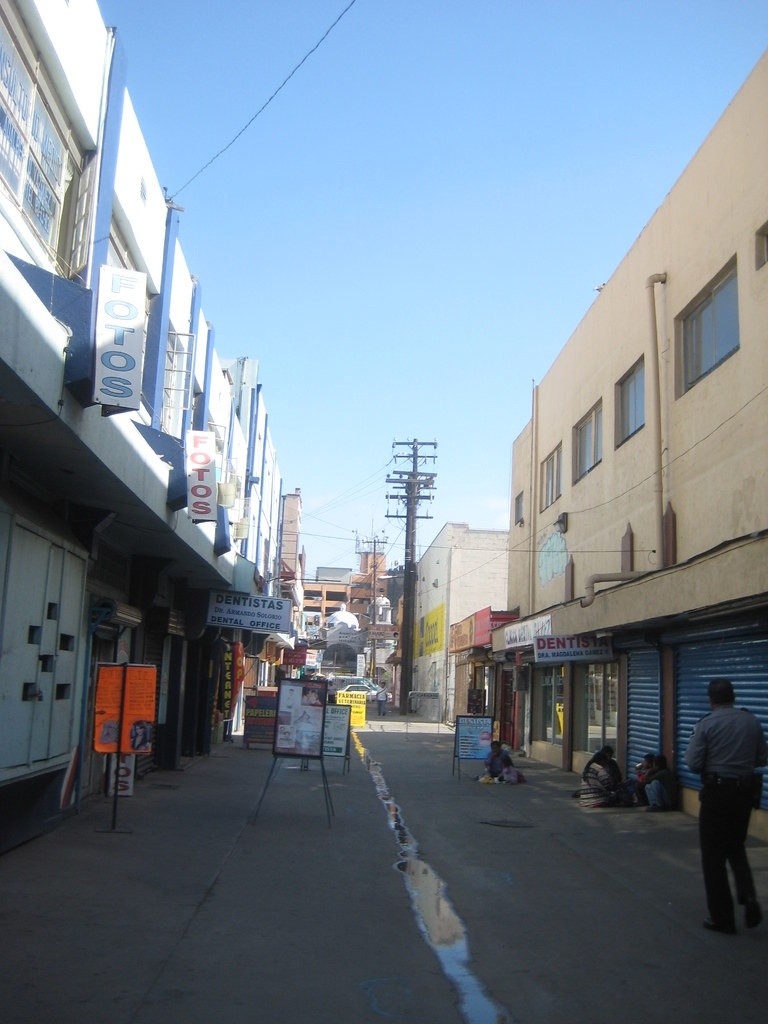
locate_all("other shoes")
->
[704,919,735,934]
[383,712,385,716]
[378,713,380,716]
[745,899,762,928]
[646,805,663,812]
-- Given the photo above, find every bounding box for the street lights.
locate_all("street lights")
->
[352,613,376,684]
[377,576,417,714]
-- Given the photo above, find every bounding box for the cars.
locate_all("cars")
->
[335,684,392,702]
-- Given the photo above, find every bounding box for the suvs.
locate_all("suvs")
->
[327,676,384,701]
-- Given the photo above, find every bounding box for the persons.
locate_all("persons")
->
[130,721,151,749]
[375,682,387,716]
[303,689,321,705]
[572,745,681,812]
[685,679,768,934]
[484,741,513,778]
